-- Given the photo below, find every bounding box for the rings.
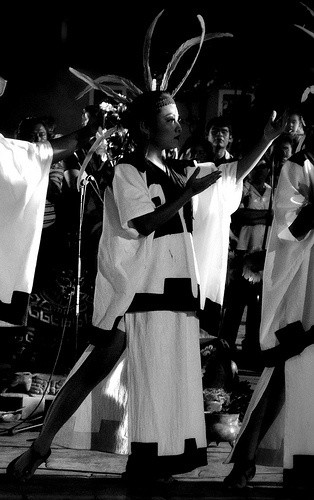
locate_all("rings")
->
[208,182,211,186]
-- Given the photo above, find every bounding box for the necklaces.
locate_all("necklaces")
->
[165,164,168,174]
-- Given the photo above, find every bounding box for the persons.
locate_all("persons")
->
[224,92,314,487]
[7,92,292,487]
[0,76,314,349]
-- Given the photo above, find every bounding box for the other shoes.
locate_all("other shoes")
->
[153,471,194,491]
[7,370,33,391]
[223,460,256,494]
